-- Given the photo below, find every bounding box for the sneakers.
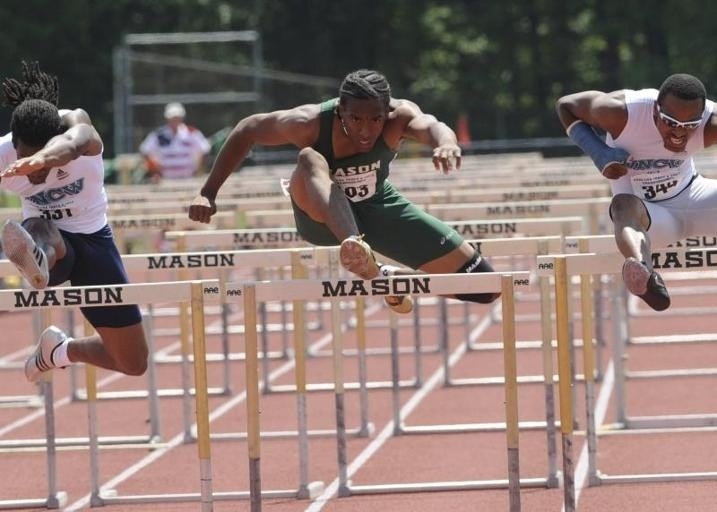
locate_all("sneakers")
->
[378,264,413,314]
[338,233,380,281]
[24,324,69,384]
[621,256,671,312]
[0,218,50,290]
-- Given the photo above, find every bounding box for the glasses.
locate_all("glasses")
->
[655,100,702,131]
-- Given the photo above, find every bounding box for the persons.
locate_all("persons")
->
[139,101,211,183]
[190,69,501,311]
[1,99,149,383]
[556,72,716,312]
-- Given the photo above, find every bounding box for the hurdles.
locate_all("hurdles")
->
[0,278,221,512]
[563,234,716,488]
[226,270,531,511]
[536,245,717,512]
[1,146,716,447]
[0,259,69,509]
[84,246,325,507]
[314,234,563,500]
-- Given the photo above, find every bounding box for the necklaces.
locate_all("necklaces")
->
[336,105,349,137]
